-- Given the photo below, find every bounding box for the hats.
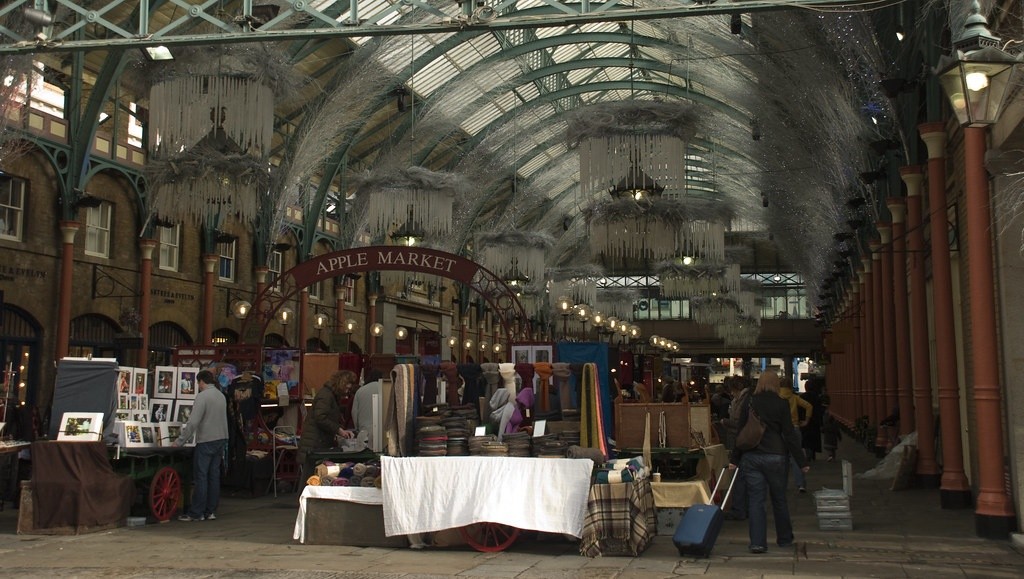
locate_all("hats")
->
[731,375,745,391]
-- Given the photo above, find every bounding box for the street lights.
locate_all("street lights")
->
[447,335,458,362]
[649,335,661,357]
[671,342,682,363]
[606,316,620,344]
[344,318,358,352]
[394,326,408,355]
[592,310,606,343]
[620,321,631,346]
[233,301,252,345]
[664,339,674,359]
[370,322,385,352]
[629,325,642,349]
[658,337,667,358]
[463,338,474,363]
[492,342,503,362]
[575,304,591,342]
[477,341,489,363]
[276,306,294,350]
[312,313,328,352]
[558,296,575,340]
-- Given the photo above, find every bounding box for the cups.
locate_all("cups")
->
[653,472,661,482]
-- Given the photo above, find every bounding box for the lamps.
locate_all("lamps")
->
[476,340,488,351]
[223,289,253,322]
[370,321,383,338]
[462,339,474,349]
[607,1,666,199]
[445,336,458,348]
[554,296,679,359]
[340,319,357,335]
[671,217,706,264]
[936,4,1024,130]
[491,343,503,353]
[499,42,529,290]
[310,306,332,330]
[394,326,409,340]
[263,304,293,325]
[389,2,427,248]
[139,44,180,65]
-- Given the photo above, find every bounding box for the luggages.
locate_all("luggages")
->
[672,464,741,558]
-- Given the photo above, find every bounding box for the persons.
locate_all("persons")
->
[661,375,759,519]
[797,381,842,462]
[729,371,809,553]
[296,371,356,502]
[779,377,812,493]
[173,370,228,521]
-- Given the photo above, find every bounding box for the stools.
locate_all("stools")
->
[268,425,302,499]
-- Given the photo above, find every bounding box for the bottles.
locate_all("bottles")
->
[42,415,49,438]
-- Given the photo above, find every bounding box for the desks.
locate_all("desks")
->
[650,478,721,539]
[578,482,648,556]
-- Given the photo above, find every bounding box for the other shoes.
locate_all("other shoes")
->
[178,513,205,522]
[205,512,215,520]
[749,544,768,553]
[827,457,832,461]
[798,485,806,492]
[778,533,794,547]
[807,454,811,462]
[812,454,815,460]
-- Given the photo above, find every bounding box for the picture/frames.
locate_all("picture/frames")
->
[159,424,183,447]
[123,421,144,447]
[150,399,174,422]
[133,368,149,394]
[178,366,200,400]
[116,366,132,393]
[114,409,131,422]
[173,400,197,424]
[140,393,149,410]
[140,425,157,444]
[129,394,138,410]
[118,394,128,409]
[181,426,200,447]
[55,410,104,442]
[132,410,150,422]
[154,366,177,399]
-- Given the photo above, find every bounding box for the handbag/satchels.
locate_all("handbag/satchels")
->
[735,393,764,451]
